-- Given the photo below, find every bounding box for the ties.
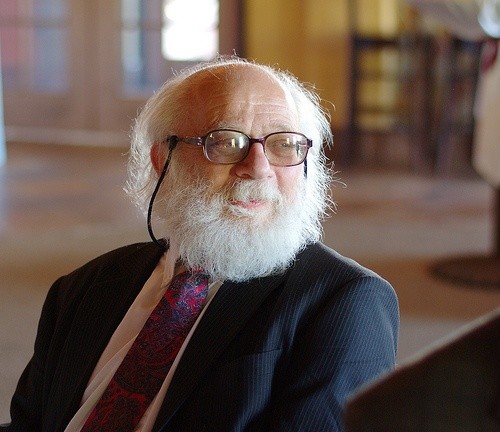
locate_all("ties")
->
[78,254,211,432]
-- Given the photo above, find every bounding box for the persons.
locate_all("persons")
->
[0,58,396,432]
[473,8,500,254]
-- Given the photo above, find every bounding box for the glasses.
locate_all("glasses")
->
[166,128,314,167]
